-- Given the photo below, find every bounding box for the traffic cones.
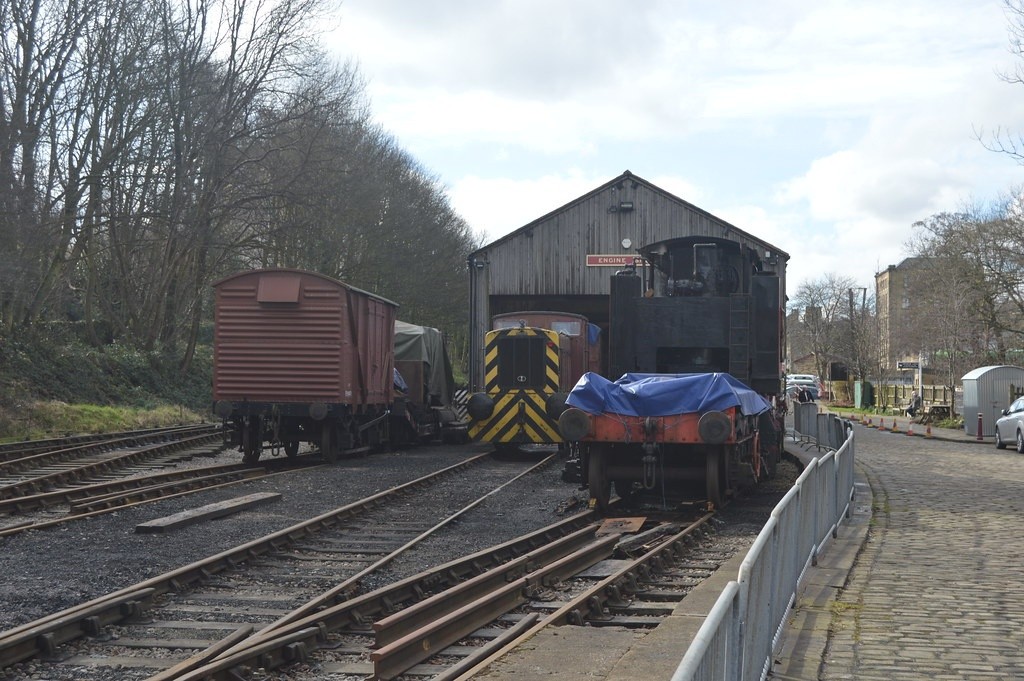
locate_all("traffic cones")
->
[858,412,863,424]
[878,417,886,431]
[868,415,875,428]
[923,421,934,439]
[906,420,915,436]
[850,409,856,421]
[862,414,868,426]
[890,418,901,433]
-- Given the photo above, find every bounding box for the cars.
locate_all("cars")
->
[785,373,822,401]
[995,396,1024,452]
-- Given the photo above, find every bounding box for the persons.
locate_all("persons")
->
[907,391,921,418]
[799,385,814,403]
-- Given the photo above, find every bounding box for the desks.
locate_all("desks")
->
[919,405,950,425]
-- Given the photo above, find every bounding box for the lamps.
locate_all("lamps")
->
[620,201,633,211]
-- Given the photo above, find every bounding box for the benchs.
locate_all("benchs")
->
[900,407,925,417]
[916,412,960,416]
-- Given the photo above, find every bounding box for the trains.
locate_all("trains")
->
[213,267,456,463]
[470,310,604,456]
[564,234,789,510]
